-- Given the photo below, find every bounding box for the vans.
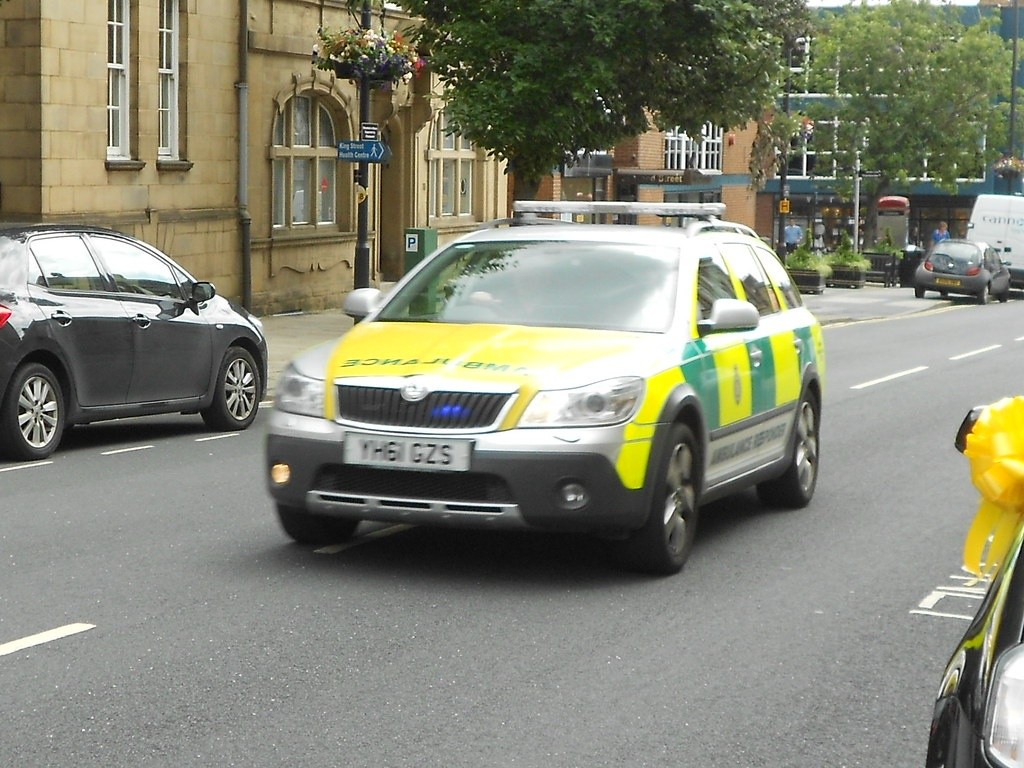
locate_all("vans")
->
[966,193,1024,295]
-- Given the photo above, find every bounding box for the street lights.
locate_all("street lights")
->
[795,31,825,176]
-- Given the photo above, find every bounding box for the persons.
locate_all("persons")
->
[783,218,802,253]
[930,221,951,250]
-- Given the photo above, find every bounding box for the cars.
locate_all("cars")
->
[914,240,1011,306]
[927,396,1023,768]
[1,221,269,465]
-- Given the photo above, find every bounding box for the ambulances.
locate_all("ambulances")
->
[262,196,827,580]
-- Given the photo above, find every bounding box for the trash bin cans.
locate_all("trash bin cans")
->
[404,226,440,316]
[900,244,926,287]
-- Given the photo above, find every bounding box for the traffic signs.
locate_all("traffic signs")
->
[338,140,385,161]
